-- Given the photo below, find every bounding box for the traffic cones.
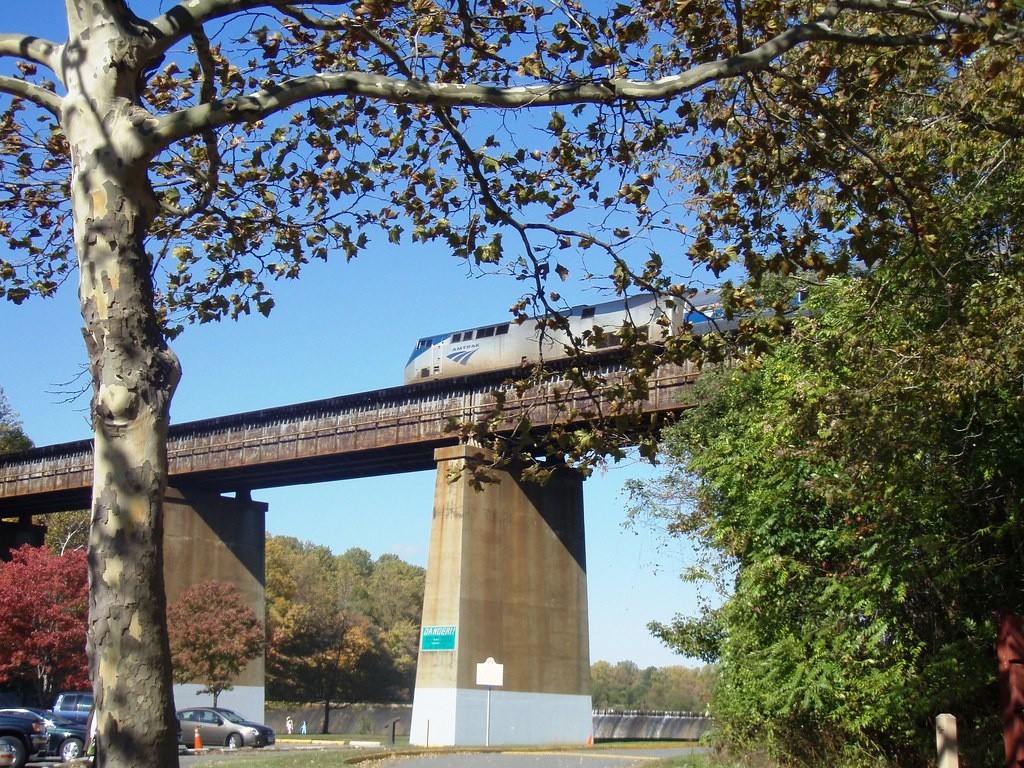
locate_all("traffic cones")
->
[194,726,203,750]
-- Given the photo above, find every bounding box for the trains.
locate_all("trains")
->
[404,273,831,386]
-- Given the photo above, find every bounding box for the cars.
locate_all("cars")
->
[0,712,50,768]
[0,707,91,768]
[177,708,275,749]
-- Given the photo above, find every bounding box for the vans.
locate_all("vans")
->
[51,692,184,744]
